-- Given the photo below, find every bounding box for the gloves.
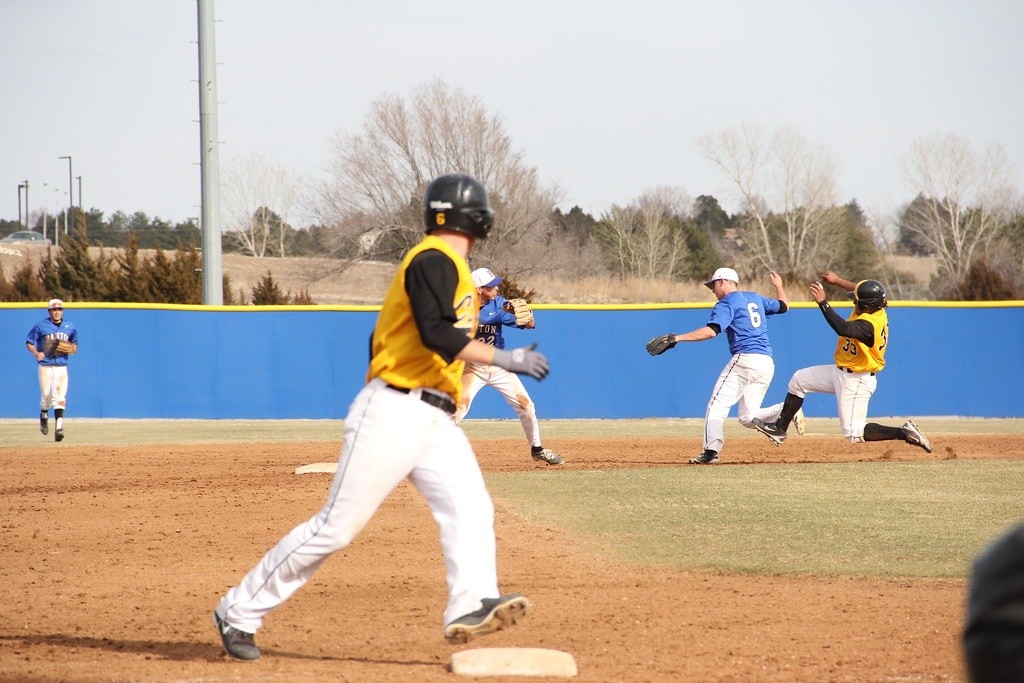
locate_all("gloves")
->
[490,342,550,381]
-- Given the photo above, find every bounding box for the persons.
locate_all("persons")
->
[26,299,78,442]
[962,528,1024,683]
[456,268,564,466]
[646,269,806,463]
[752,271,933,453]
[214,175,550,660]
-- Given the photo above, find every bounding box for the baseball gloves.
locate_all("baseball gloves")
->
[645,333,677,357]
[501,298,533,329]
[55,338,77,355]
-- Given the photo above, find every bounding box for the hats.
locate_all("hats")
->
[48,298,64,310]
[704,268,739,290]
[471,268,505,288]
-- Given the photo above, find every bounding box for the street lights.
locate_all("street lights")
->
[75,176,81,212]
[18,180,29,231]
[58,156,72,238]
[42,183,68,246]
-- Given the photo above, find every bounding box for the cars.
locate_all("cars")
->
[0,231,52,247]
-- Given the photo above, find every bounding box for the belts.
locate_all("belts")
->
[386,384,457,415]
[838,366,874,376]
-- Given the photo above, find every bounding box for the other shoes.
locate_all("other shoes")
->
[55,430,64,441]
[40,414,48,435]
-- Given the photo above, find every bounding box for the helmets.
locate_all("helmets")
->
[423,174,495,240]
[846,279,885,311]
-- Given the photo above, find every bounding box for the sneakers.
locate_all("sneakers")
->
[532,449,565,465]
[688,453,719,464]
[902,420,932,453]
[792,406,806,435]
[752,417,788,445]
[213,610,260,662]
[443,595,528,645]
